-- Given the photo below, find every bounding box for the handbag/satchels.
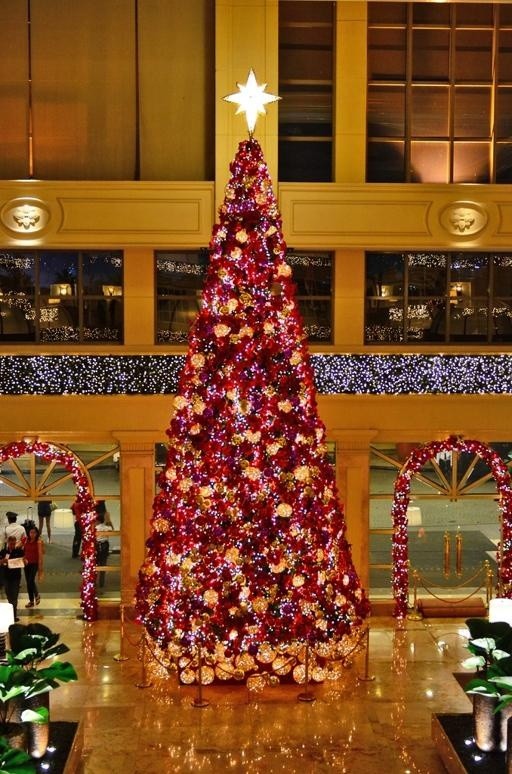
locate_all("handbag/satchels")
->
[49,503,58,511]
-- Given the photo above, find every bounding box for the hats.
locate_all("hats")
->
[6,512,18,517]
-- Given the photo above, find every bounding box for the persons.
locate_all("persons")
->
[69,494,84,559]
[94,498,114,590]
[36,484,53,545]
[23,526,45,608]
[1,536,25,623]
[1,511,28,551]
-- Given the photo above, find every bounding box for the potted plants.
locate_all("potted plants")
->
[0,625,78,774]
[463,618,512,774]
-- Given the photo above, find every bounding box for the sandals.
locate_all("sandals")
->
[25,593,40,607]
